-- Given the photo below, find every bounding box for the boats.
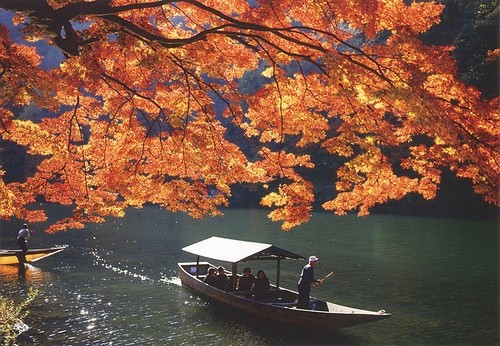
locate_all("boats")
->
[0,245,70,265]
[177,236,392,330]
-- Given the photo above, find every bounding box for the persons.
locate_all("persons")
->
[238,268,255,291]
[252,270,269,291]
[204,267,238,292]
[297,256,323,309]
[18,224,30,252]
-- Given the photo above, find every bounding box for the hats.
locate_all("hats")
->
[309,256,318,262]
[217,266,226,272]
